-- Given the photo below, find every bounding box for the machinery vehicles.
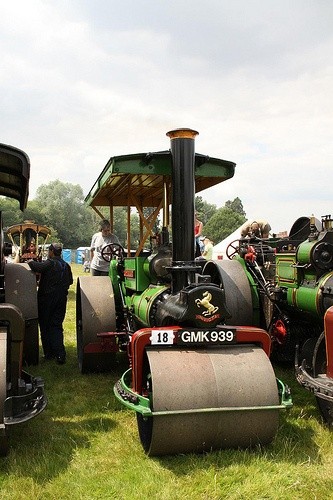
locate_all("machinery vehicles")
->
[75,126,294,457]
[0,142,53,459]
[225,211,333,426]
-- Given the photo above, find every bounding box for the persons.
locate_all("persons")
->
[89,219,121,278]
[25,242,73,366]
[240,221,272,241]
[193,209,204,259]
[14,229,44,292]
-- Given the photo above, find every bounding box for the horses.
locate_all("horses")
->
[194,290,219,316]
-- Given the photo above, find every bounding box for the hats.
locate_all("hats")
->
[47,243,62,253]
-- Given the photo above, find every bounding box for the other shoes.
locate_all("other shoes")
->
[57,350,66,362]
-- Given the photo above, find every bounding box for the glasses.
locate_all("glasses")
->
[103,229,110,232]
